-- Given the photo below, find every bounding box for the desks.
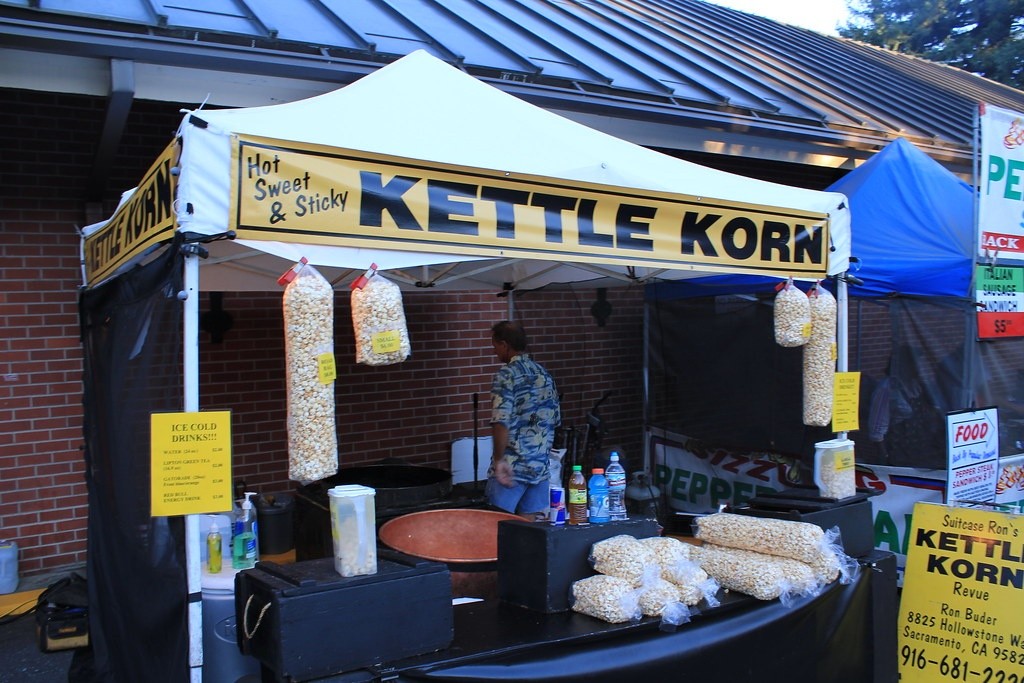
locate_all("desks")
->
[241,545,898,683]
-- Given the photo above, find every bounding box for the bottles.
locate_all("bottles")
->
[605,451,627,520]
[588,468,609,523]
[570,465,587,525]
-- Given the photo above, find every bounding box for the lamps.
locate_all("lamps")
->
[589,288,613,328]
[198,292,235,343]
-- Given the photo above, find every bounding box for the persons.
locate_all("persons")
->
[486,321,560,523]
[868,343,946,468]
[937,338,993,408]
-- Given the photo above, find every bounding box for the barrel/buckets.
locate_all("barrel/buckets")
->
[0,539,19,594]
[253,492,294,554]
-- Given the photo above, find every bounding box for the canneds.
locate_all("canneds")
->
[549,487,565,525]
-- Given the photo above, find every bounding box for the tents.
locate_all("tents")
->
[79,48,852,683]
[644,138,981,435]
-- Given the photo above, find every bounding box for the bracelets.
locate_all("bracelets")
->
[885,454,888,457]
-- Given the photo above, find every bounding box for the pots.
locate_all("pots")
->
[312,464,452,509]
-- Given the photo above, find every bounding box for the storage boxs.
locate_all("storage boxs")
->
[234,549,453,683]
[720,486,875,563]
[495,514,660,611]
[31,608,91,654]
